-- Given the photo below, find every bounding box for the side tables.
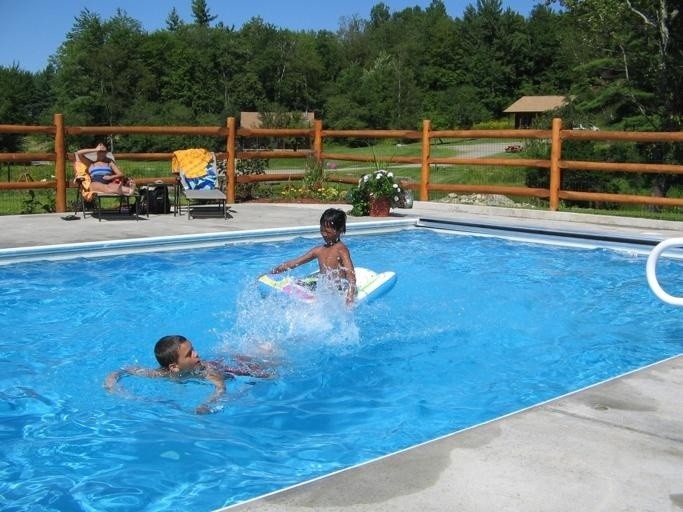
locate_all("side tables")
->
[146,181,177,218]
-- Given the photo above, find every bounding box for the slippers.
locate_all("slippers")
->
[59,215,81,221]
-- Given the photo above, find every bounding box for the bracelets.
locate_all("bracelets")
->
[112,174,115,179]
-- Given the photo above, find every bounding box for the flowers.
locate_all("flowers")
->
[344,141,405,217]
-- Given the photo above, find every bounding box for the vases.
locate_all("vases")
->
[368,192,390,217]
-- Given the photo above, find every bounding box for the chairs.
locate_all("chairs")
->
[173,148,227,220]
[71,147,140,222]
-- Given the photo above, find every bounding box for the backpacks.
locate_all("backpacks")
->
[131,183,170,214]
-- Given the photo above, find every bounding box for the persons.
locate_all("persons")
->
[102,335,289,415]
[76,140,136,198]
[255,207,357,306]
